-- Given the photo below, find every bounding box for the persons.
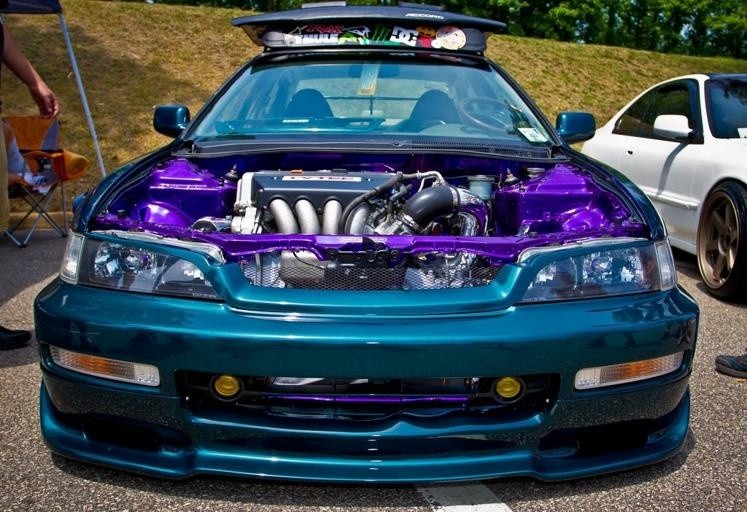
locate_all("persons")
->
[0,18,63,351]
[0,118,38,236]
[714,353,746,381]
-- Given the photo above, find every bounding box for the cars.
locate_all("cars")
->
[577,70,744,308]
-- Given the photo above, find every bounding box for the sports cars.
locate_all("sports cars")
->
[30,0,704,488]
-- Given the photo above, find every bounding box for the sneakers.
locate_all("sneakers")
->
[715,353,746,378]
[0,326,31,350]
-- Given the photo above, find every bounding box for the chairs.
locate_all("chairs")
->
[0,114,90,249]
[281,89,461,124]
